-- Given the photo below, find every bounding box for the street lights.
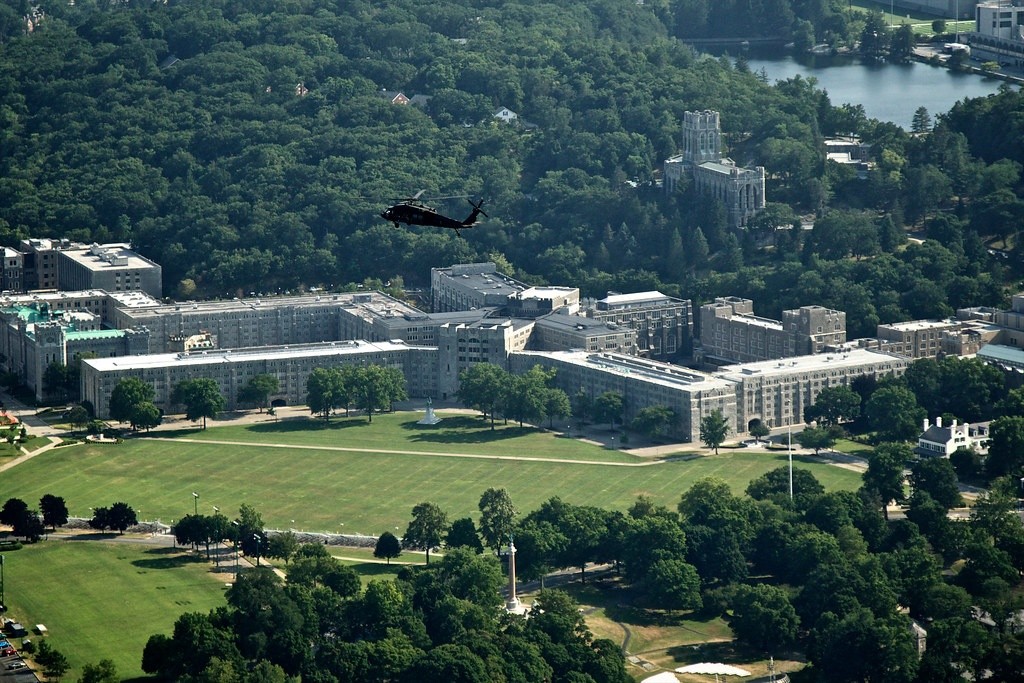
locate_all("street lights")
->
[253,533,261,567]
[192,492,199,551]
[231,520,239,577]
[213,506,220,567]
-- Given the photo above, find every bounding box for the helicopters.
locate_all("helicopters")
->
[349,190,488,238]
